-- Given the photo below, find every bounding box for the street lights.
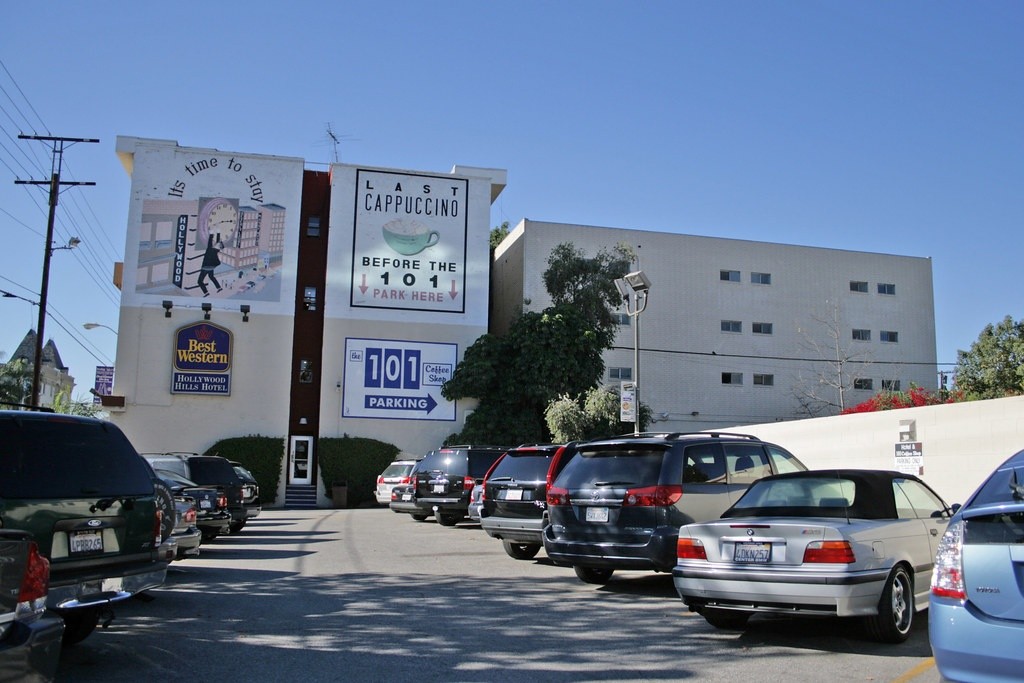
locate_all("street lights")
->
[31,235,81,412]
[613,271,653,439]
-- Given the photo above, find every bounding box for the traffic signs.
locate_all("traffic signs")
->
[350,168,469,314]
[342,337,458,421]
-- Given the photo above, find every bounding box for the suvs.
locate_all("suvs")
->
[541,431,813,585]
[477,442,579,561]
[141,451,262,534]
[0,402,179,646]
[372,444,519,526]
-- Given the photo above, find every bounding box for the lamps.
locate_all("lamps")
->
[240,305,251,321]
[202,303,211,320]
[83,323,117,336]
[163,300,173,318]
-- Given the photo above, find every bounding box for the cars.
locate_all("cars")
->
[0,528,66,683]
[672,468,960,644]
[151,469,233,561]
[926,448,1024,683]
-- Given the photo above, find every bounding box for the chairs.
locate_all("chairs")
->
[821,498,850,508]
[788,494,815,507]
[735,456,755,472]
[692,463,716,485]
